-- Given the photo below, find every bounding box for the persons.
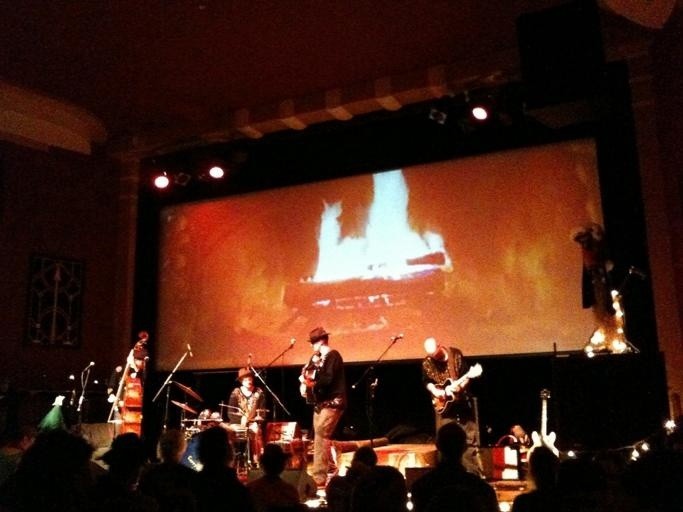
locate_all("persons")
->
[422,342,480,446]
[304,326,347,490]
[1,421,309,512]
[320,424,498,510]
[510,426,681,510]
[227,367,268,469]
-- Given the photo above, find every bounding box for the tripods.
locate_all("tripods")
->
[239,419,258,474]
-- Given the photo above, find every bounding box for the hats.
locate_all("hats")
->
[235,367,255,381]
[310,327,329,344]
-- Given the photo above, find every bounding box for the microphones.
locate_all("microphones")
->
[290,336,296,346]
[187,343,193,357]
[391,334,404,340]
[85,361,95,370]
[247,353,252,364]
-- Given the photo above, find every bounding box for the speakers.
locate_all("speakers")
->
[247,469,317,503]
[406,466,437,491]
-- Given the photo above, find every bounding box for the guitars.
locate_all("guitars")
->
[305,351,320,404]
[526,389,559,463]
[431,363,482,415]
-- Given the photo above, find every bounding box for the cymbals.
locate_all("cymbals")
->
[173,381,202,402]
[170,400,197,415]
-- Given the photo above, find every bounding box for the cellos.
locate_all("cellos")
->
[118,332,148,438]
